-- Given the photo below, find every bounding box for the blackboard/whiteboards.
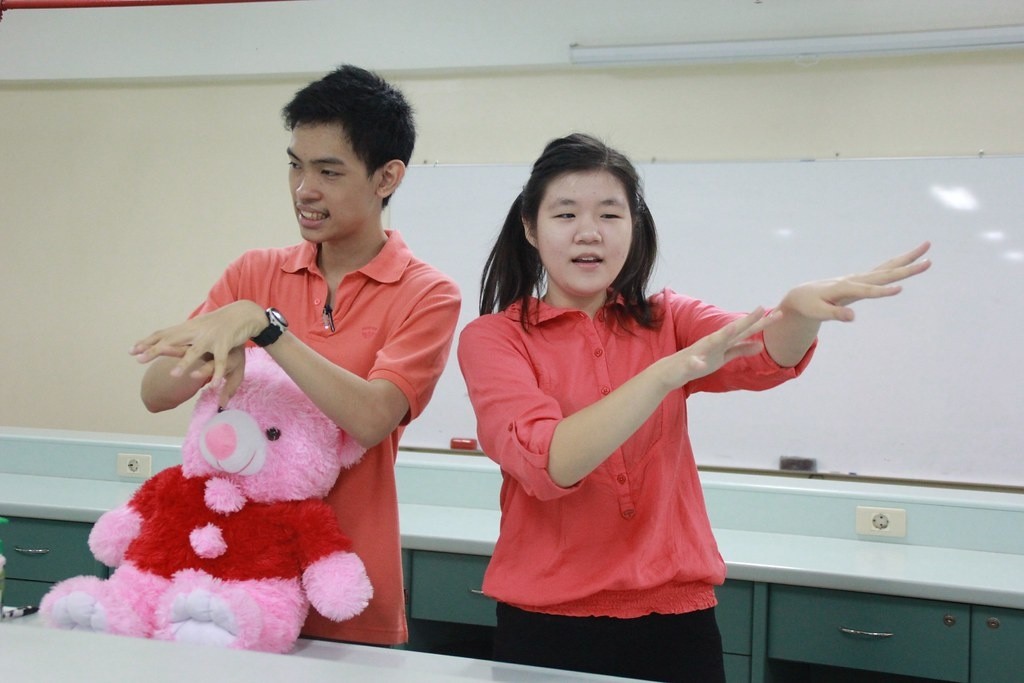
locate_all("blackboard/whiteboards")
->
[387,154,1024,494]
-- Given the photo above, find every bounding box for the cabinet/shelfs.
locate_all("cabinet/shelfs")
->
[0,434,1024,683]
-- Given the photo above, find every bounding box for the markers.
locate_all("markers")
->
[829,471,857,475]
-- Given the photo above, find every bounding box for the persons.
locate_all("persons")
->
[458,133,932,682]
[131,64,463,650]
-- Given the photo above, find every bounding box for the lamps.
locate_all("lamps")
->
[568,24,1024,73]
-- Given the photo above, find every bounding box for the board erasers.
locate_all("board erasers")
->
[779,455,817,472]
[449,437,477,450]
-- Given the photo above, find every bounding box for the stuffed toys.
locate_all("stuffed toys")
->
[37,347,373,653]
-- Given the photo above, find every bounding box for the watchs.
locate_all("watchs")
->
[249,306,289,347]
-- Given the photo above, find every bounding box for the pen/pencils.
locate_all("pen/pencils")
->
[3,605,39,618]
